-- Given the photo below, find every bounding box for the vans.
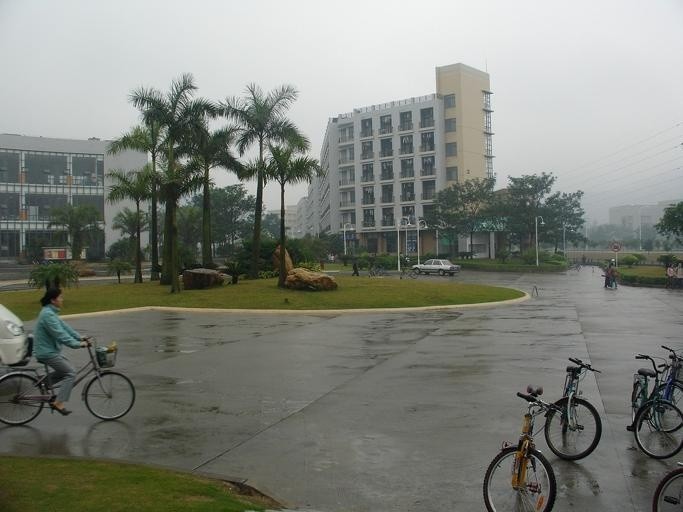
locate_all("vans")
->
[0,304,34,372]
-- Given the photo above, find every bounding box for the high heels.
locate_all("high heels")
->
[49,402,72,417]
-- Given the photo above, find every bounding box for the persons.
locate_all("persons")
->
[663,261,683,290]
[605,265,617,289]
[31,287,95,415]
[352,264,359,276]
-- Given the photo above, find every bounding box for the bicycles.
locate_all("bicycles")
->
[651,461,683,512]
[399,266,419,279]
[0,335,137,426]
[628,352,683,460]
[482,382,557,511]
[645,343,683,434]
[542,357,604,461]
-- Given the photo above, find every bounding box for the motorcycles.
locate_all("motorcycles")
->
[600,273,617,288]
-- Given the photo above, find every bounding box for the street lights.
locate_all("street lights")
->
[415,218,428,266]
[396,214,412,273]
[534,213,546,267]
[343,221,354,266]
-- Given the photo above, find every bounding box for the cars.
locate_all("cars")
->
[410,258,462,276]
